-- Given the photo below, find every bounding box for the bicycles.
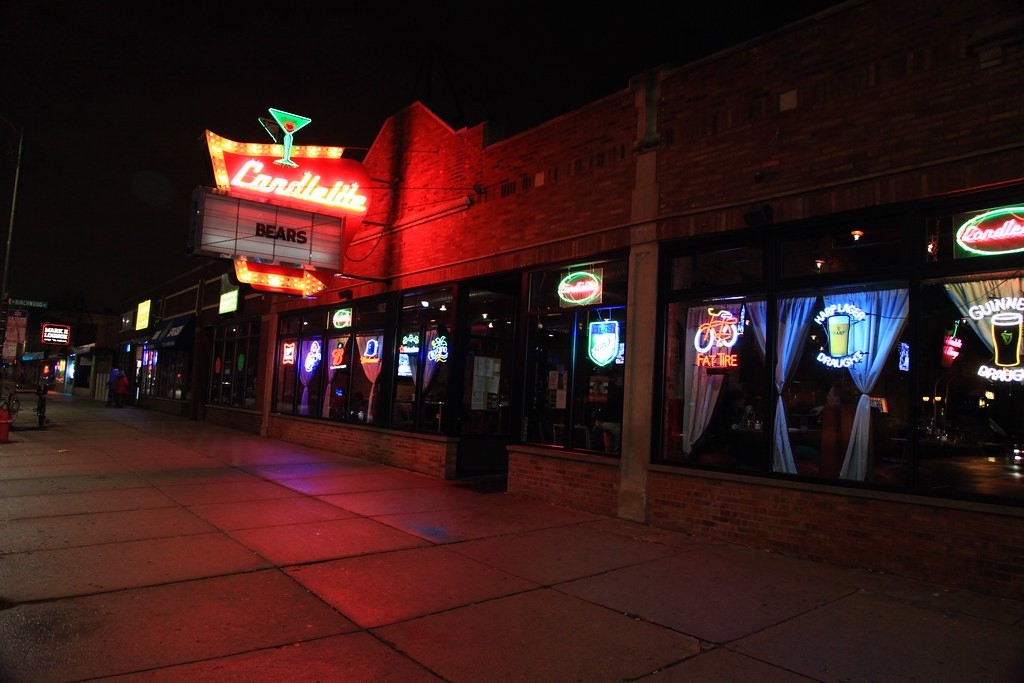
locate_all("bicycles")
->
[30,382,57,428]
[1,384,22,423]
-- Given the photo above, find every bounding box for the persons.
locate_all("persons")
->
[349,392,369,425]
[112,370,129,408]
[792,384,851,463]
[105,364,120,408]
[710,389,754,474]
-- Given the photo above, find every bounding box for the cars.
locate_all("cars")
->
[1010,442,1024,461]
